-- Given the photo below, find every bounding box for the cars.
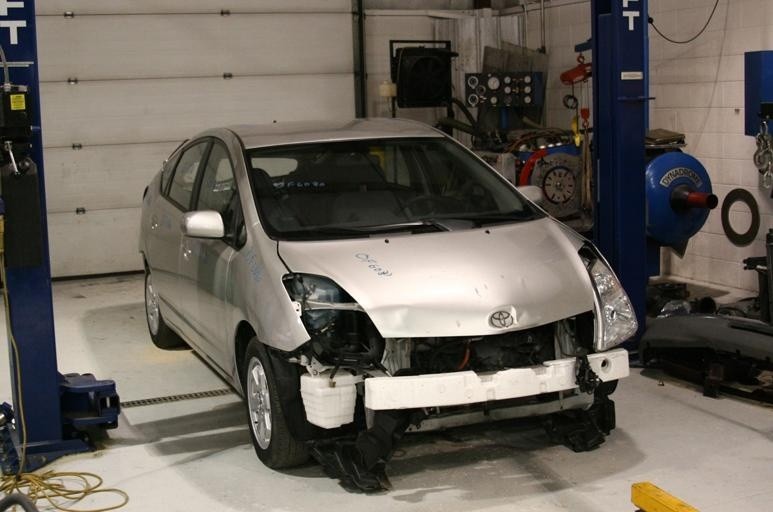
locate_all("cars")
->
[138,115,641,494]
